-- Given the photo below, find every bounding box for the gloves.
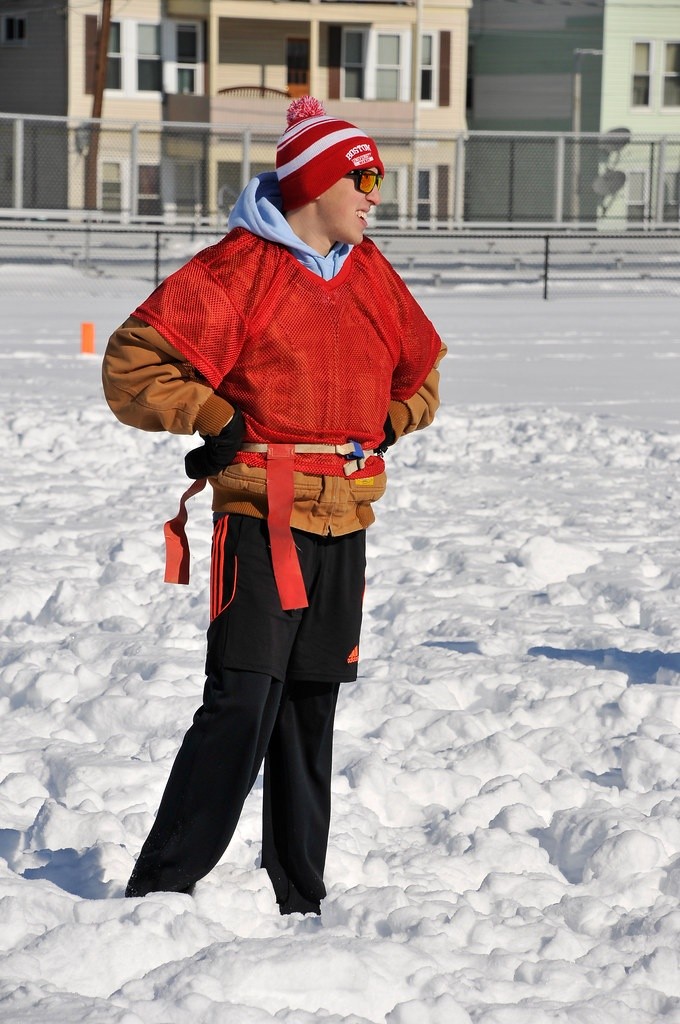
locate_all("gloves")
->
[184,407,248,480]
[377,413,398,453]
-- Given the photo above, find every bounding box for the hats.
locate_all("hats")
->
[275,95,385,211]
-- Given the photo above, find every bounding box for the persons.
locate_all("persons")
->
[101,98,447,915]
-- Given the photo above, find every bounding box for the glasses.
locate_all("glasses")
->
[345,169,383,193]
